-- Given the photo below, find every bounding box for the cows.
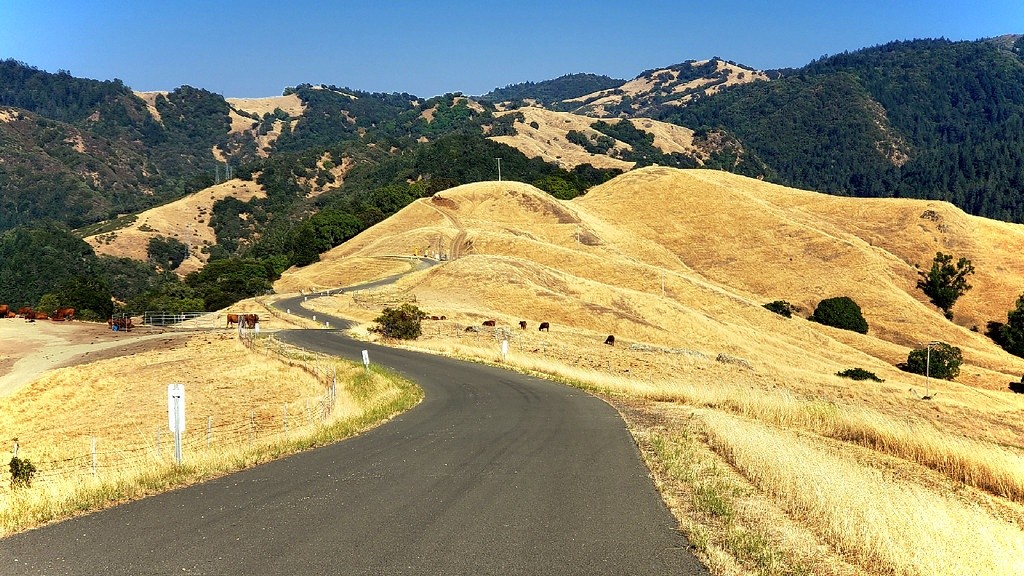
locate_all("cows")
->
[482,320,496,326]
[421,315,446,320]
[519,321,528,329]
[226,313,259,328]
[0,304,76,321]
[108,317,135,329]
[604,335,615,346]
[539,322,550,332]
